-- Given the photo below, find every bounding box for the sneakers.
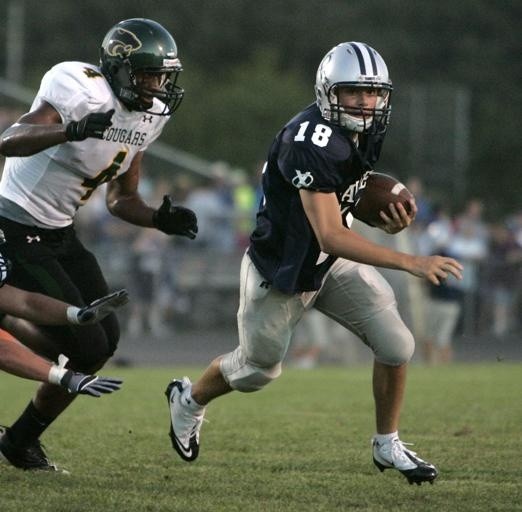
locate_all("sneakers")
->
[371,436,439,487]
[0,424,72,475]
[164,376,210,462]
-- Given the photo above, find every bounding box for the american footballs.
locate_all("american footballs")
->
[351,173,415,224]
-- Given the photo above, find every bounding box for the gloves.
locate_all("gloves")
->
[65,108,116,142]
[152,193,199,240]
[59,369,123,399]
[76,288,131,327]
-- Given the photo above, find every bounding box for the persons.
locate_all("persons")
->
[0,231,130,398]
[1,18,199,475]
[165,40,463,485]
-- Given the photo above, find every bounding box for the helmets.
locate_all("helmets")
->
[98,17,185,117]
[313,40,394,137]
